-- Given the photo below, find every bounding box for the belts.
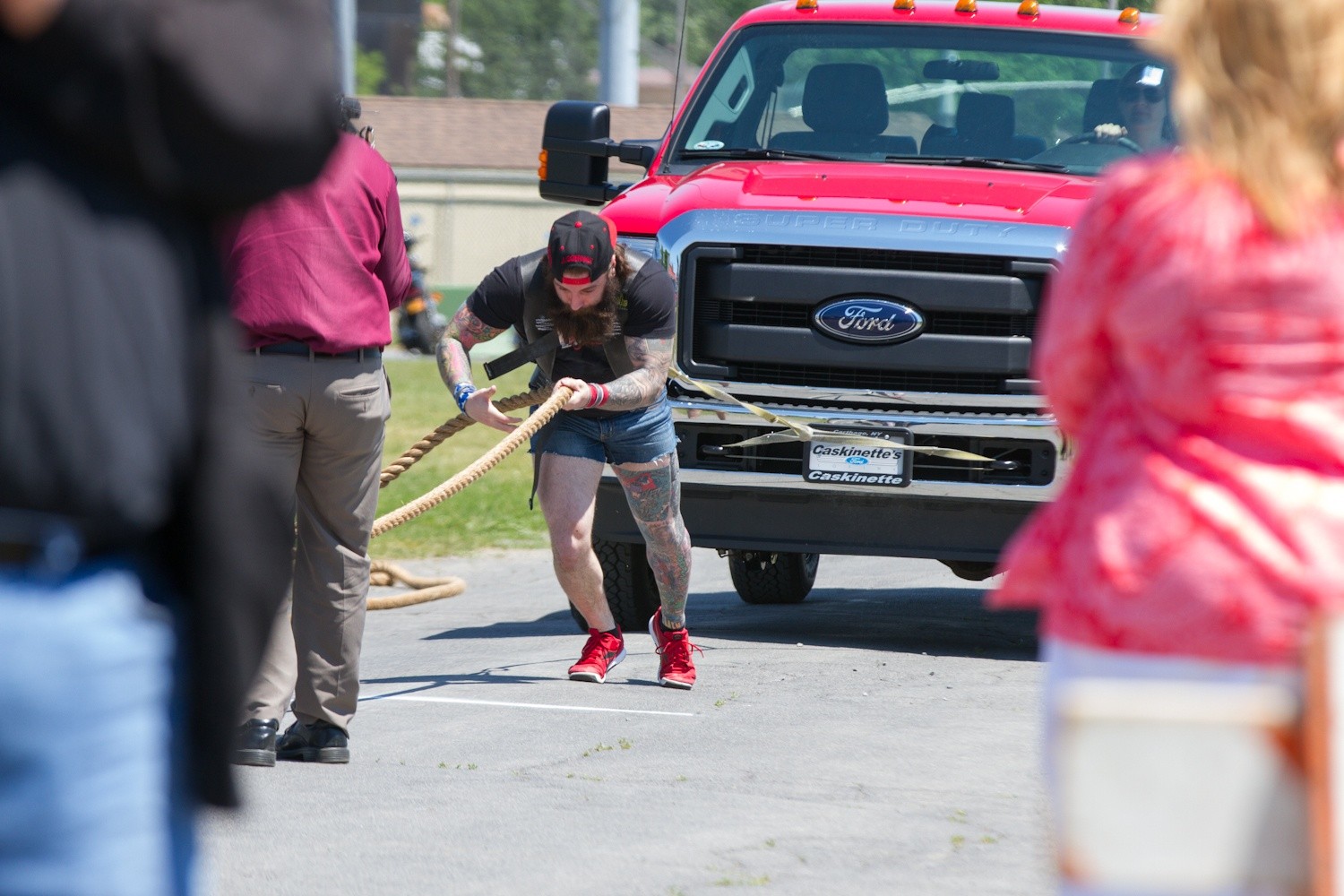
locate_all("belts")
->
[245,342,382,359]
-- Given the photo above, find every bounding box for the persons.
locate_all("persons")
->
[433,209,698,692]
[1095,62,1183,160]
[986,0,1344,896]
[0,0,343,896]
[221,132,411,768]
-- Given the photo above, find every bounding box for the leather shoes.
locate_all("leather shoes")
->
[233,718,279,765]
[276,718,351,763]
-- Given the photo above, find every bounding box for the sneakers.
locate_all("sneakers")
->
[568,624,627,683]
[648,605,704,688]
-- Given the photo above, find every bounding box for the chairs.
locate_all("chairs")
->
[767,62,1120,162]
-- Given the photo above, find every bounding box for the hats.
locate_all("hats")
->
[547,210,614,286]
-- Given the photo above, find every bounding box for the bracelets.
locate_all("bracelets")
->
[584,382,609,410]
[450,378,477,420]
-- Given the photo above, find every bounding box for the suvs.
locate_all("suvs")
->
[535,0,1182,634]
